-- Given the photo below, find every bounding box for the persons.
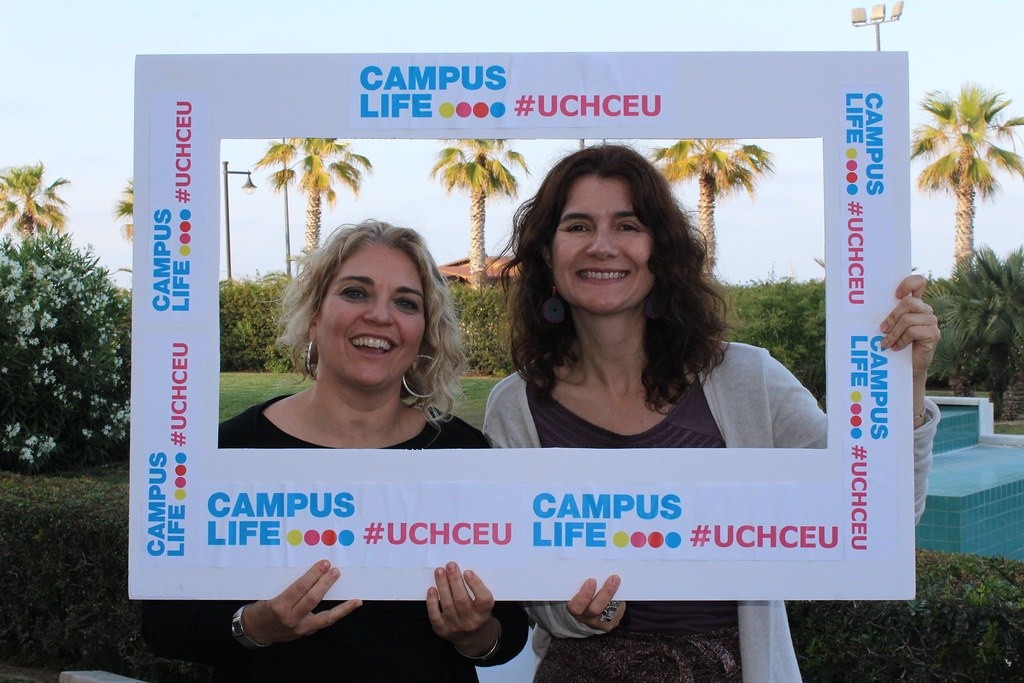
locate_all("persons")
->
[479,143,942,683]
[140,221,530,683]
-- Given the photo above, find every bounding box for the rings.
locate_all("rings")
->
[600,600,622,623]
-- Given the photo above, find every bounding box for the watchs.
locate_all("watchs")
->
[231,604,271,647]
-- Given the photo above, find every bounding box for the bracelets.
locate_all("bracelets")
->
[913,404,927,420]
[455,630,500,660]
